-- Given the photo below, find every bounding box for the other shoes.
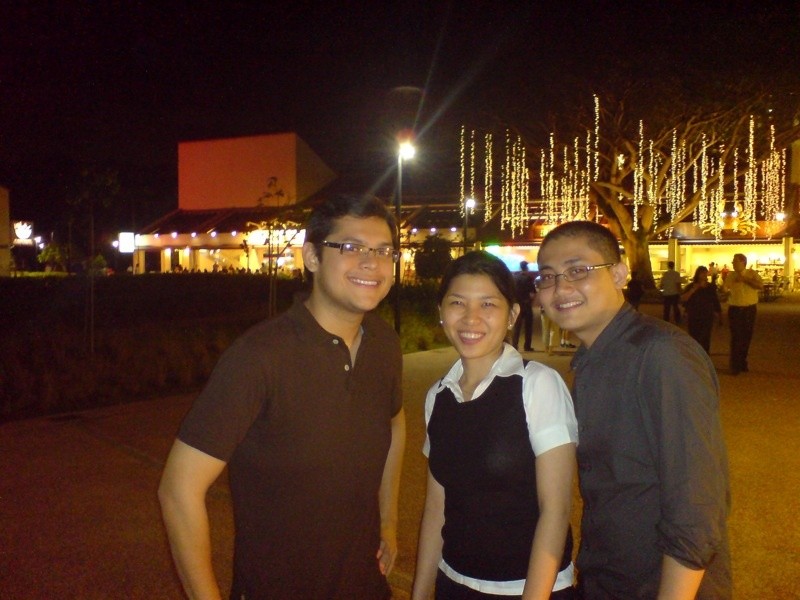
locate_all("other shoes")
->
[561,343,565,347]
[729,367,741,375]
[548,351,555,355]
[677,319,682,323]
[566,343,576,348]
[524,345,534,351]
[742,364,748,371]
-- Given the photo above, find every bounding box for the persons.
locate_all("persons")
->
[408,250,580,600]
[533,220,735,600]
[678,266,721,355]
[708,261,800,294]
[157,192,407,600]
[723,253,763,376]
[656,260,690,328]
[628,271,643,312]
[512,260,576,350]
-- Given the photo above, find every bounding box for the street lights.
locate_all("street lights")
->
[395,126,419,339]
[464,198,476,256]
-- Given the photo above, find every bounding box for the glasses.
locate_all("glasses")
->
[321,241,400,263]
[532,263,613,288]
